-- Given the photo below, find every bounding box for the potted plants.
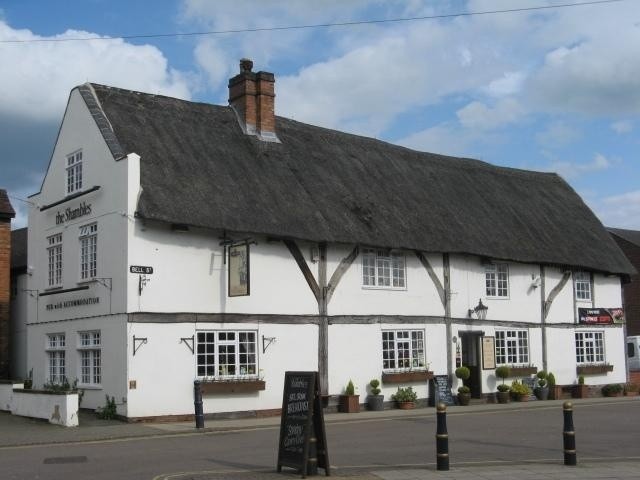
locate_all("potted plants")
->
[455,366,472,406]
[496,366,563,404]
[602,382,639,398]
[340,378,417,414]
[577,372,585,398]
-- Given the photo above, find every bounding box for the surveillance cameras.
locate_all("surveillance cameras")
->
[533,278,541,288]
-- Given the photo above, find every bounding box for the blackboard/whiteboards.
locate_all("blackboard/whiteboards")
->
[523,378,537,401]
[433,375,453,405]
[278,372,316,469]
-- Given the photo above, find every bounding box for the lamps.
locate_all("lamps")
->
[468,297,489,321]
[531,272,542,290]
